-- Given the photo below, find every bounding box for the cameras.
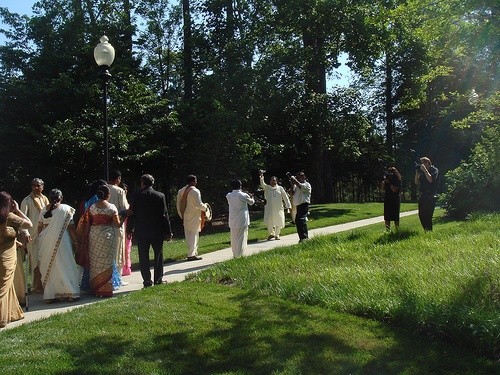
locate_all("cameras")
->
[286,172,294,182]
[258,169,266,174]
[413,161,420,169]
[383,171,393,176]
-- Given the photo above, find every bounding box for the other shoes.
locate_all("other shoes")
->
[275,237,280,240]
[188,256,202,261]
[267,235,274,240]
[162,281,167,284]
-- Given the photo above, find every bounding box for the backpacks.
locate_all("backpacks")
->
[431,167,448,194]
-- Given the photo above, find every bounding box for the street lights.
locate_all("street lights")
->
[95,35,115,183]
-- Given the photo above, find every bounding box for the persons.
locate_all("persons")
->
[287,171,300,225]
[226,179,255,259]
[118,181,135,276]
[125,174,173,288]
[176,174,213,261]
[84,179,128,293]
[37,188,84,304]
[381,167,402,234]
[415,157,440,233]
[103,169,131,287]
[18,177,57,295]
[6,200,34,307]
[289,170,312,244]
[258,168,292,241]
[74,184,128,299]
[0,190,34,328]
